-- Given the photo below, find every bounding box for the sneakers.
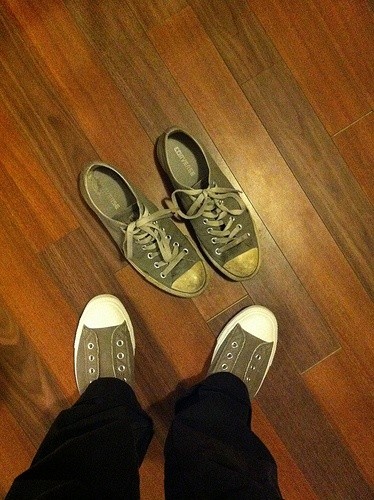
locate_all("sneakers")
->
[156,127,262,281]
[74,294,135,397]
[78,161,209,298]
[204,304,278,402]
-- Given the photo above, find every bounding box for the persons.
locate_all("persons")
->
[5,295,285,500]
